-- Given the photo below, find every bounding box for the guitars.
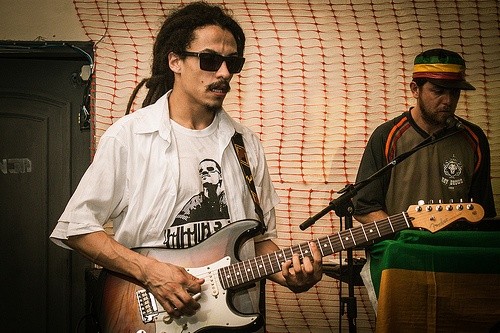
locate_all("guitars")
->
[95,198,485,333]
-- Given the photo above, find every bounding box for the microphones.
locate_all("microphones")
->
[444,116,467,130]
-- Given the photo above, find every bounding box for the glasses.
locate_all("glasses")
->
[184,52,245,74]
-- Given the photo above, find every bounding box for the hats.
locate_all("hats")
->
[412,49,476,90]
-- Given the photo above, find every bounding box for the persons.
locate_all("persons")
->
[49,1,322,333]
[353,49,497,258]
